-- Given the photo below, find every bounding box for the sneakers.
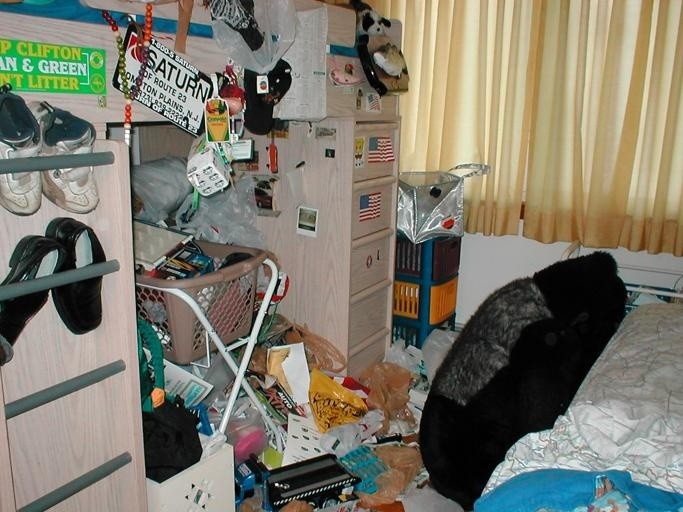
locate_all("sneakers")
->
[26,101,98,213]
[0,82,41,216]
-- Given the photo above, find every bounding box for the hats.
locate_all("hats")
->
[242,59,292,135]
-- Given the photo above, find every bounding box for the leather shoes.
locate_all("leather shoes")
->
[44,218,105,334]
[0,235,66,365]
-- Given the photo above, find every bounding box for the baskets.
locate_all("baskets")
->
[133,240,266,367]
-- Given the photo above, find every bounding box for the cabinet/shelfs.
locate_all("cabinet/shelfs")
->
[0,0,458,511]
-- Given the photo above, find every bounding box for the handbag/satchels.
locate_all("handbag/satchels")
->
[395,162,488,246]
[140,390,202,484]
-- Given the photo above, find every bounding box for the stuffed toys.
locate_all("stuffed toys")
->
[348,1,413,98]
